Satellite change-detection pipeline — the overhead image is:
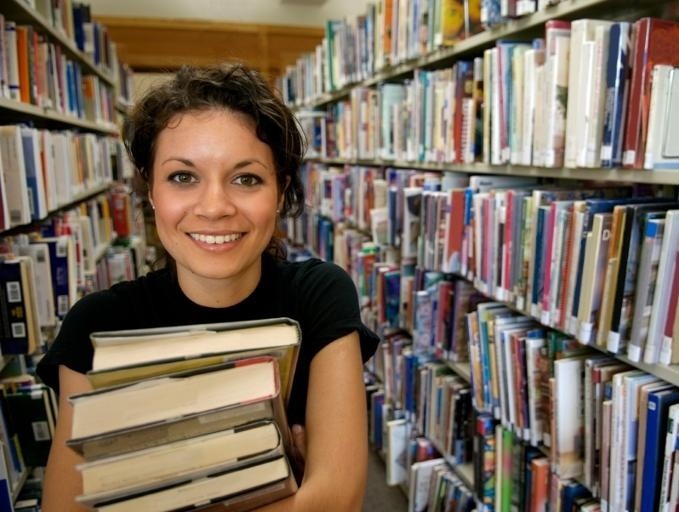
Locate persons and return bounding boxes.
[35,68,380,512]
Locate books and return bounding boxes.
[294,15,679,172]
[291,160,678,369]
[0,16,113,125]
[269,1,581,109]
[84,237,134,299]
[0,188,119,358]
[0,349,57,512]
[0,122,115,230]
[65,317,302,512]
[364,374,479,512]
[478,370,676,512]
[0,0,113,77]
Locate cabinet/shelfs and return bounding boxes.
[0,0,150,512]
[277,0,679,512]
